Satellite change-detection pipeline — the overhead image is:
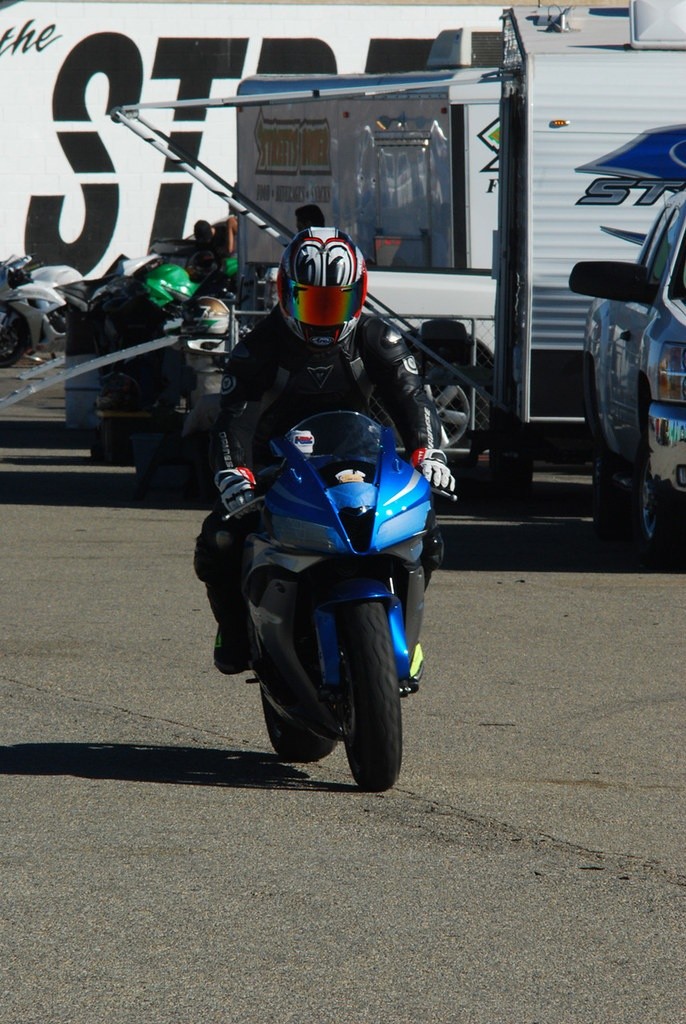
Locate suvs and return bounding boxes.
[567,189,686,557]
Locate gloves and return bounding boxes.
[215,469,261,519]
[414,448,456,494]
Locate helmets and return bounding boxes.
[277,227,368,348]
[145,263,199,306]
[191,293,231,334]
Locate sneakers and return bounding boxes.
[213,622,251,675]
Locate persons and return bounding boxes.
[195,228,456,701]
[296,203,323,233]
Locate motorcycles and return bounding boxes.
[0,254,89,369]
[230,409,434,789]
